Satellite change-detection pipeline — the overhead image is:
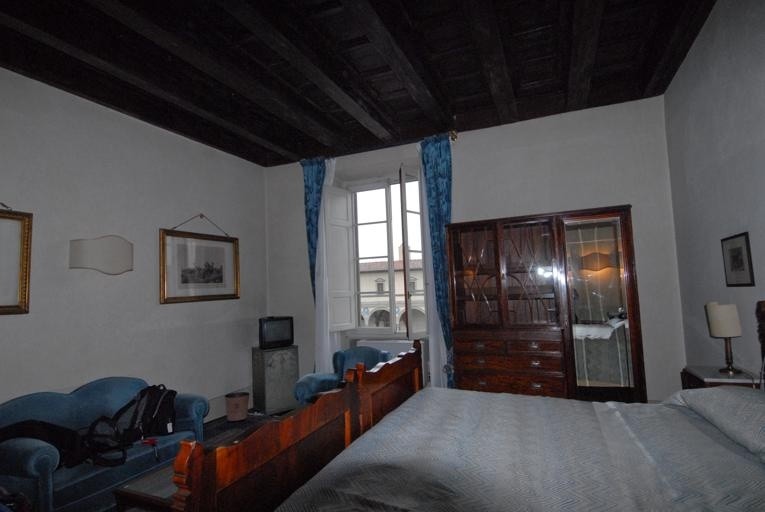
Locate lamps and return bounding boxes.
[707,302,744,374]
[68,233,135,276]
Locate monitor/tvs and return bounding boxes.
[258,315,294,351]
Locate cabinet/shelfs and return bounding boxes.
[443,201,650,404]
[252,346,300,416]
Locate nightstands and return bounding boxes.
[679,364,761,392]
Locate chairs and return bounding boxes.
[292,346,392,400]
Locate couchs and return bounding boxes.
[1,375,211,512]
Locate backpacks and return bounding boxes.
[114,384,177,436]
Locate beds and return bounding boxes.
[170,300,765,512]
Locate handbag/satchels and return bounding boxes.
[0,416,126,467]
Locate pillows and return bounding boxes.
[664,382,765,460]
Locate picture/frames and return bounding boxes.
[720,231,755,288]
[159,229,242,302]
[0,209,33,316]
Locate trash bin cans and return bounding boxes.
[225,392,249,423]
